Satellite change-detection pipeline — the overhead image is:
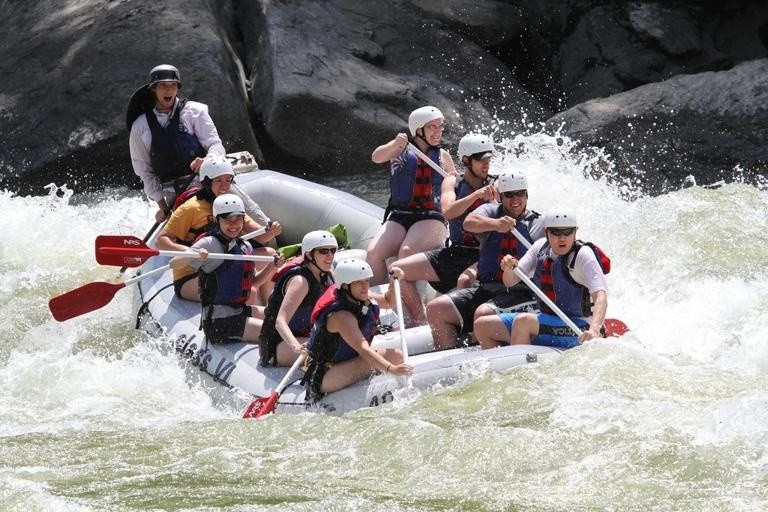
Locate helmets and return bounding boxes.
[213,194,245,220]
[498,171,528,193]
[301,230,338,261]
[334,259,374,290]
[148,64,183,90]
[543,205,577,228]
[408,106,445,138]
[459,134,494,162]
[199,156,234,182]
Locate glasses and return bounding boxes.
[425,124,444,131]
[315,248,336,255]
[503,190,526,198]
[548,228,574,236]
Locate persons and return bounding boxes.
[426,170,546,351]
[387,134,499,325]
[475,206,608,351]
[366,106,455,284]
[154,156,281,305]
[258,231,338,367]
[170,194,284,342]
[305,259,414,393]
[130,64,278,251]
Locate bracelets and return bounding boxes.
[387,363,394,373]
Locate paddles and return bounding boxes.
[243,341,305,419]
[47,228,265,322]
[95,234,277,267]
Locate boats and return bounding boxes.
[132,170,571,411]
